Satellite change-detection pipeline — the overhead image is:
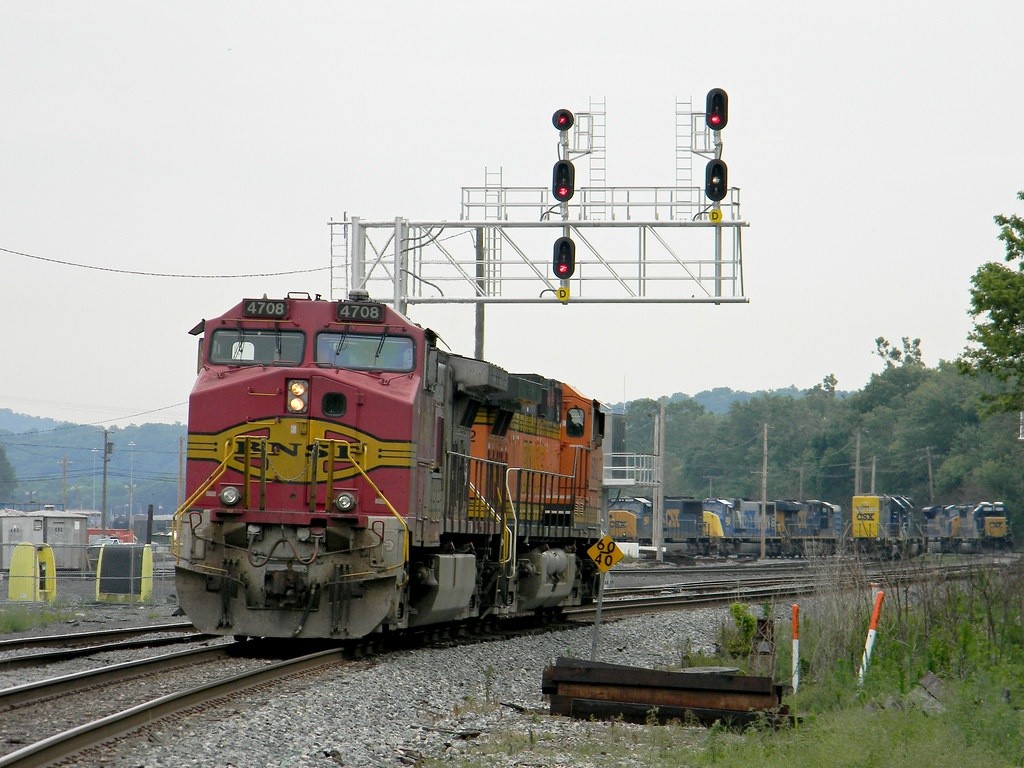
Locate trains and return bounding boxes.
[167,285,612,662]
[608,490,1011,555]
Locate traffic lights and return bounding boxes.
[551,108,574,131]
[705,87,729,132]
[552,160,575,202]
[552,237,575,279]
[706,159,728,202]
